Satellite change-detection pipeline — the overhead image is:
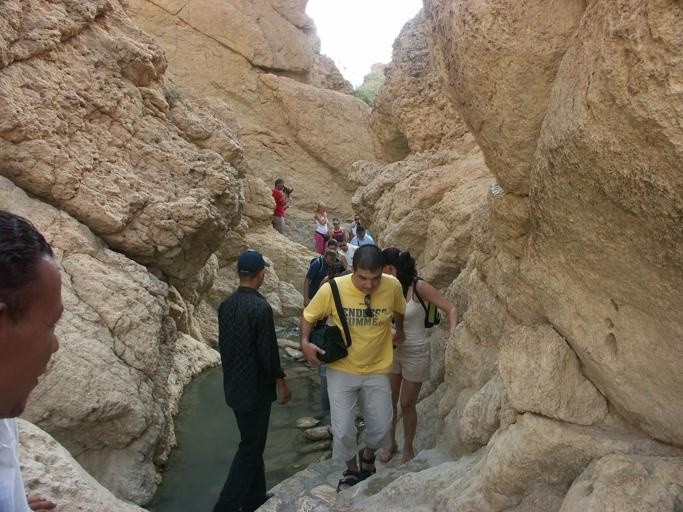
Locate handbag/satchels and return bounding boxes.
[324,235,330,241]
[424,301,441,329]
[307,324,348,365]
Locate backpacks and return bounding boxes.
[311,256,339,276]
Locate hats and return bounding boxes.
[236,250,269,276]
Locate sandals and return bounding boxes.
[401,447,413,466]
[381,440,399,463]
[358,449,378,482]
[336,466,359,497]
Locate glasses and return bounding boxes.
[364,294,373,318]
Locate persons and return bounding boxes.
[211,250,290,512]
[300,245,408,494]
[271,179,290,238]
[380,247,459,464]
[302,213,375,309]
[314,202,329,255]
[1,210,64,511]
